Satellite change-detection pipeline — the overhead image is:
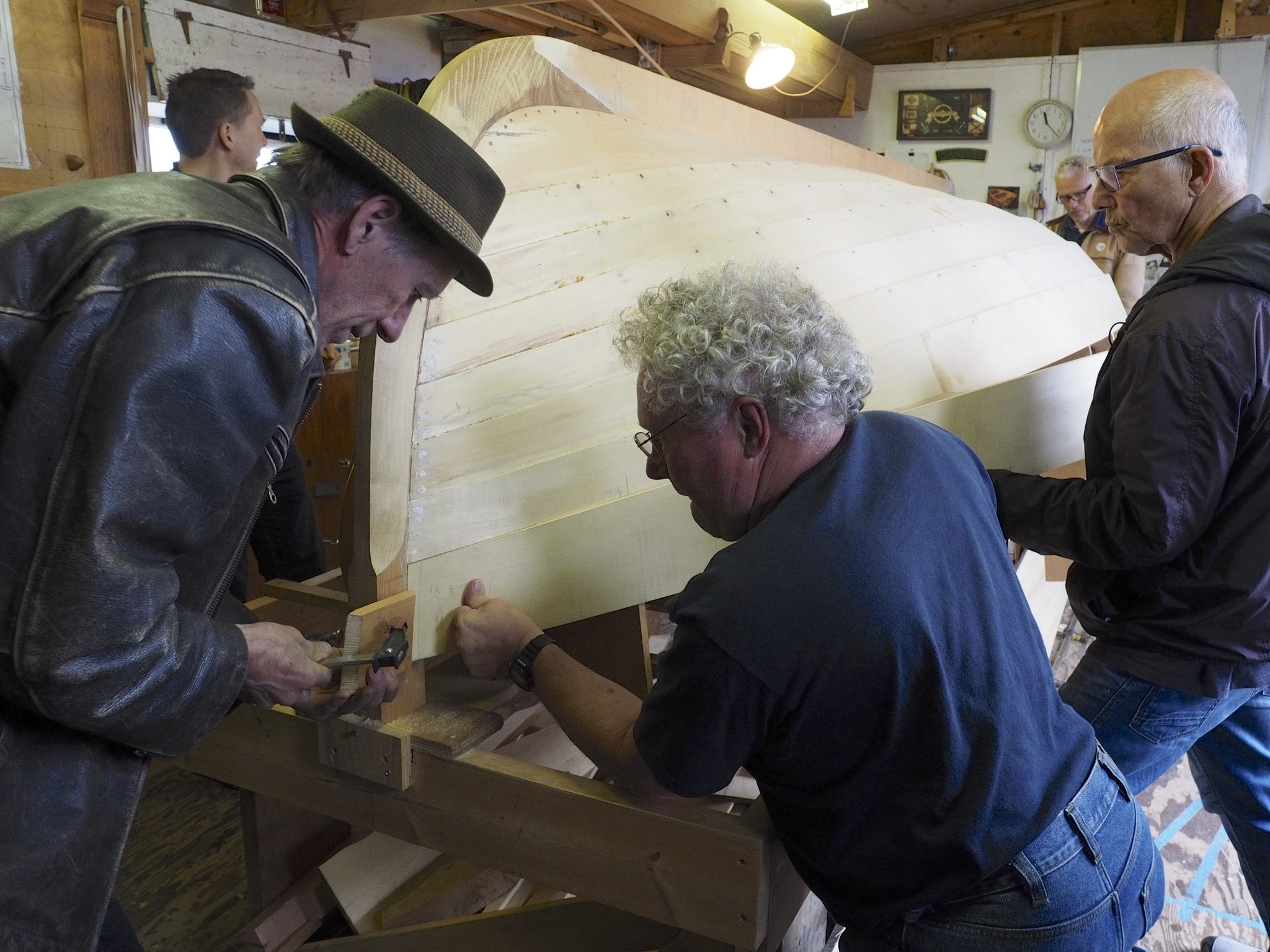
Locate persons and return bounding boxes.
[165,64,334,604]
[984,65,1270,942]
[1,91,509,951]
[1044,155,1146,317]
[447,257,1168,952]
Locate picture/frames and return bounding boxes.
[896,87,991,140]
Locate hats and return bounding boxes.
[290,86,506,298]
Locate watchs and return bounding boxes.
[508,633,561,693]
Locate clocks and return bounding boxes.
[1022,99,1072,150]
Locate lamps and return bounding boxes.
[743,32,798,90]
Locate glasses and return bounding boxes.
[1056,184,1092,204]
[1085,145,1223,193]
[634,393,759,465]
